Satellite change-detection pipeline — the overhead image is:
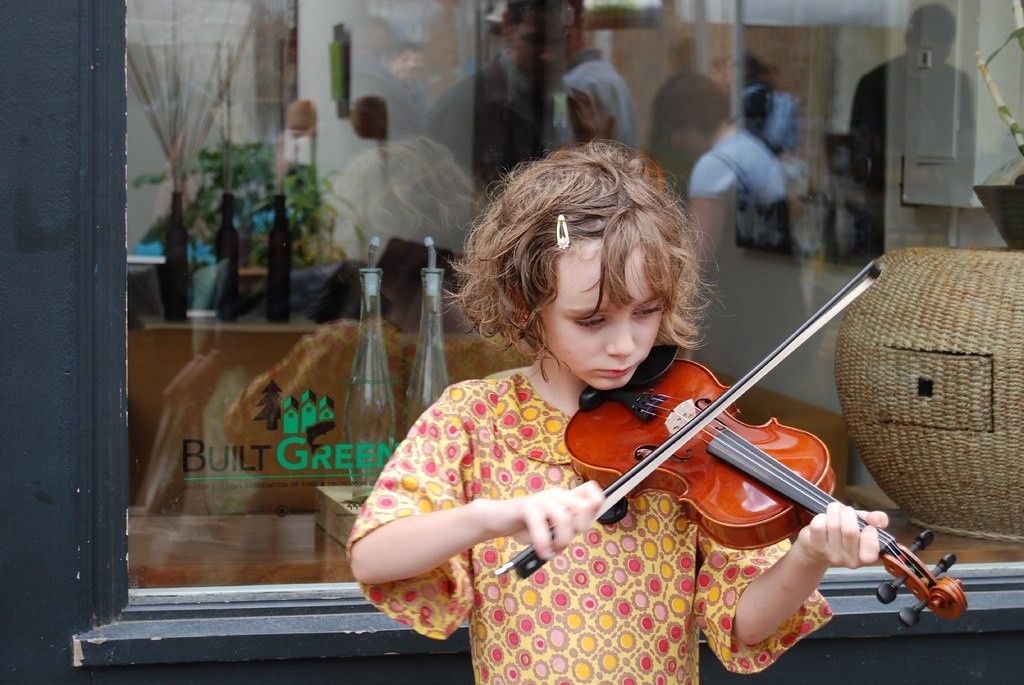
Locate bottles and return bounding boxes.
[403,237,449,438]
[342,235,396,504]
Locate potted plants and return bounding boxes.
[123,0,291,323]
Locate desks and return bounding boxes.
[129,314,351,514]
[314,486,372,560]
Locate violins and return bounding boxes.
[563,357,968,629]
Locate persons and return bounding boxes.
[844,6,975,264]
[347,139,888,685]
[346,0,805,281]
